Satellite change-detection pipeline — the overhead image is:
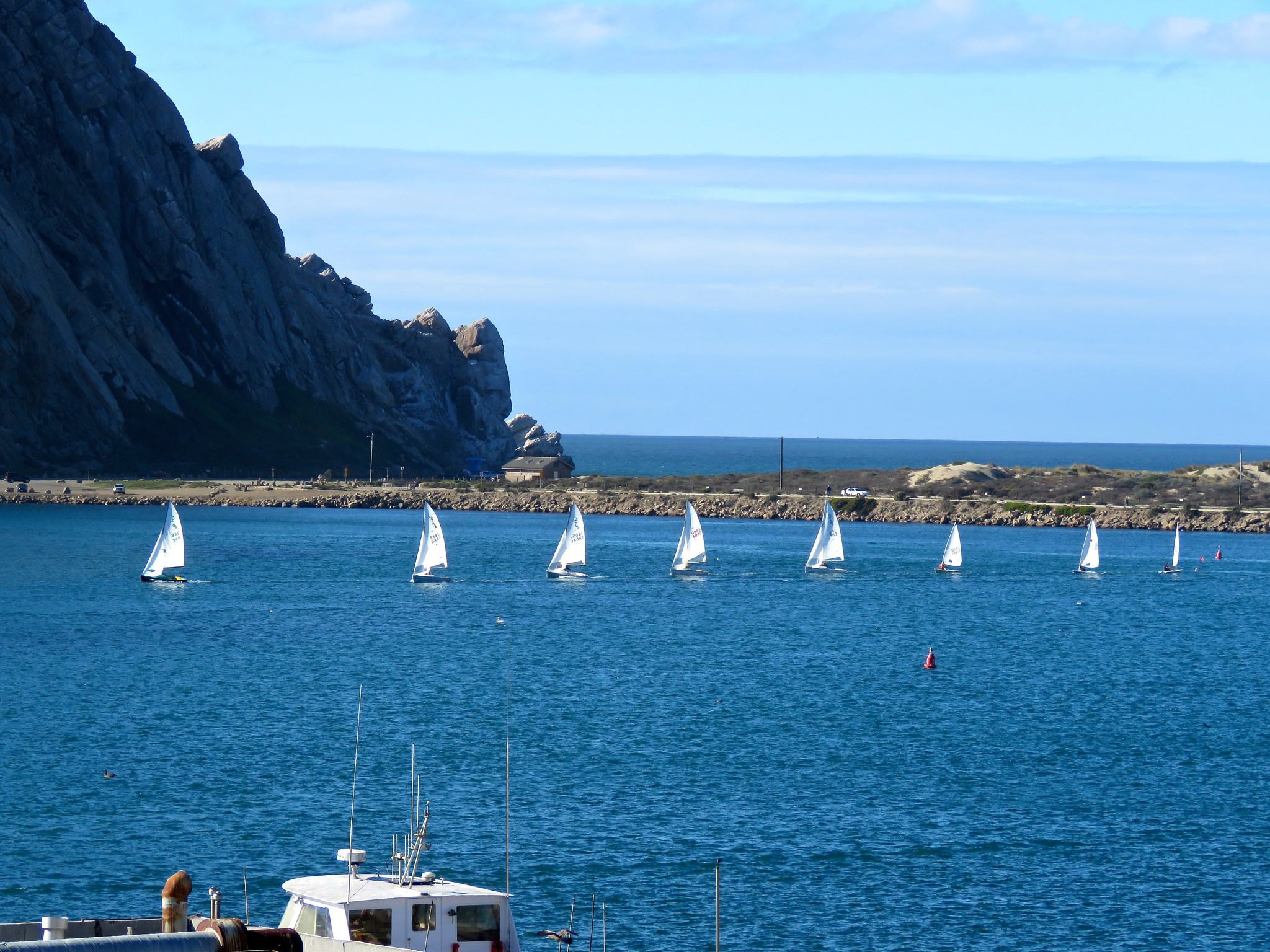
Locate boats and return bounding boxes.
[272,683,524,952]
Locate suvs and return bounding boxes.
[113,484,126,494]
[5,471,31,483]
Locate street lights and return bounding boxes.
[776,437,783,491]
[1236,448,1242,504]
[367,433,374,482]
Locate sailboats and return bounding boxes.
[934,522,962,573]
[670,499,709,576]
[805,500,847,573]
[1072,518,1106,573]
[1158,522,1183,573]
[546,502,588,578]
[412,501,452,583]
[141,501,188,582]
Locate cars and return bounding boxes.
[16,483,28,492]
[471,470,506,480]
[841,487,868,497]
